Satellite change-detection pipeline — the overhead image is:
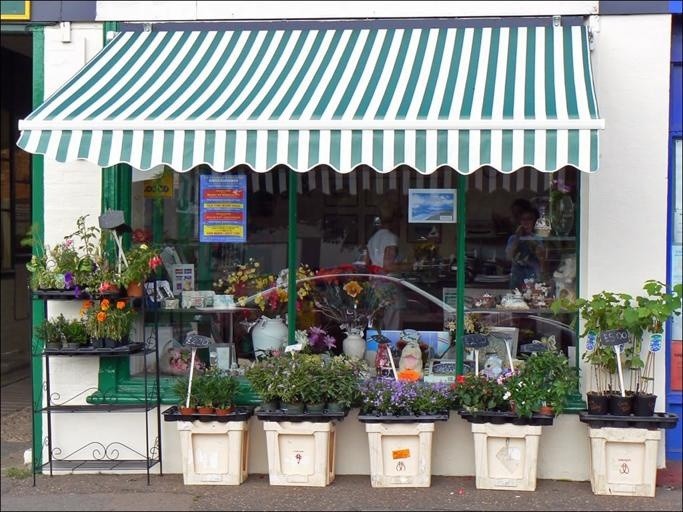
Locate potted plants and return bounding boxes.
[457,281,683,416]
[173,369,241,413]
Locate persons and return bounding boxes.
[505,198,556,291]
[365,207,419,330]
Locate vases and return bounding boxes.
[343,330,365,357]
[45,337,127,351]
[250,313,287,357]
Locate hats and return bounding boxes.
[99,211,132,233]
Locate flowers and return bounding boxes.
[211,256,395,334]
[17,215,164,343]
[246,350,452,414]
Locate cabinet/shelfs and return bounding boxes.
[32,287,162,484]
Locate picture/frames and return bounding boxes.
[318,179,444,248]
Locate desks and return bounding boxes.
[143,303,259,372]
